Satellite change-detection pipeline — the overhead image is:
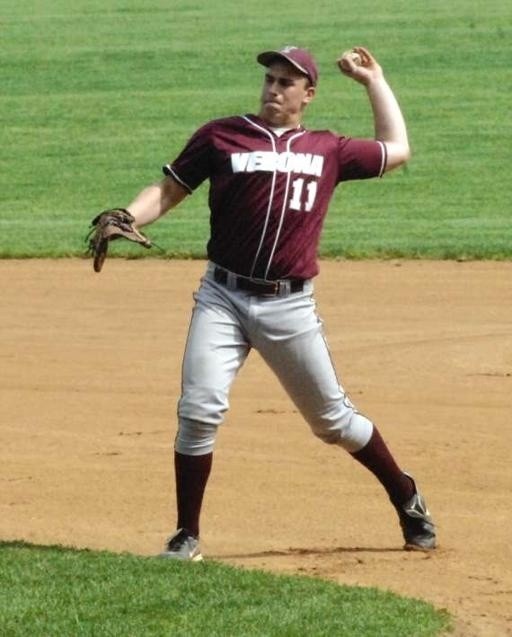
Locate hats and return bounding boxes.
[256,45,319,87]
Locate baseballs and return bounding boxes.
[338,50,365,77]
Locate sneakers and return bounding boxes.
[157,527,203,562]
[388,471,439,550]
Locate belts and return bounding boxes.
[213,266,304,297]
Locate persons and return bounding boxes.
[88,43,438,564]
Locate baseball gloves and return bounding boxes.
[86,208,152,272]
